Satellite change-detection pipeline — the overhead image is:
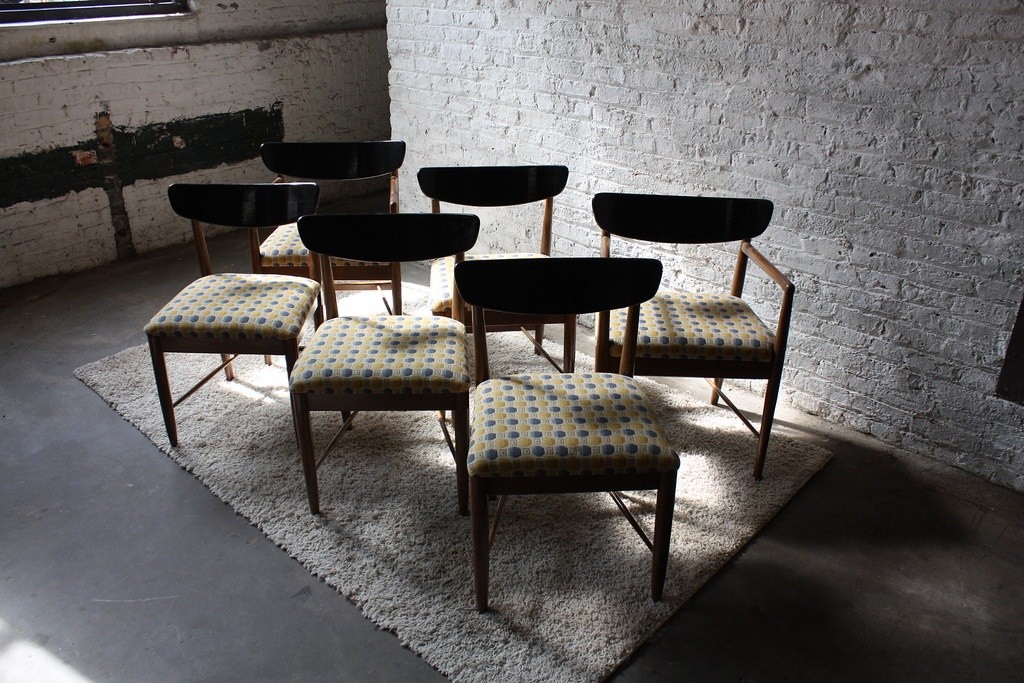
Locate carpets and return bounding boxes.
[72,273,834,683]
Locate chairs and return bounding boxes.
[590,192,795,479]
[144,182,353,453]
[289,213,480,516]
[418,164,577,376]
[249,140,406,314]
[452,257,681,613]
